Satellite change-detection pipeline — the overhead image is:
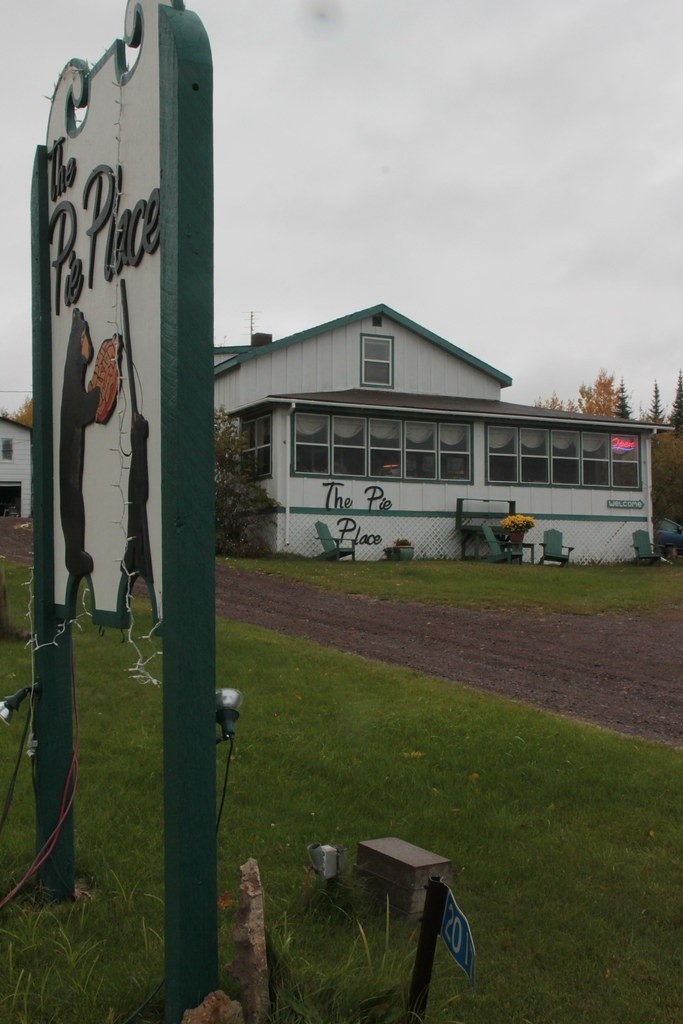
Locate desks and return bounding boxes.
[459,530,512,561]
[500,543,534,564]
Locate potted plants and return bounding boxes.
[383,538,415,560]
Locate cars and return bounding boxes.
[653,515,683,558]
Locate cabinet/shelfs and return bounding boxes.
[455,497,515,530]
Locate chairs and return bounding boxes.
[314,520,357,562]
[538,528,575,567]
[630,529,662,567]
[479,525,523,565]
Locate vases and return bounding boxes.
[508,532,524,543]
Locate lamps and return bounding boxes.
[0,681,42,726]
[382,455,400,468]
[214,687,244,740]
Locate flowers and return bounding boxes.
[500,514,536,534]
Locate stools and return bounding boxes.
[356,837,453,919]
